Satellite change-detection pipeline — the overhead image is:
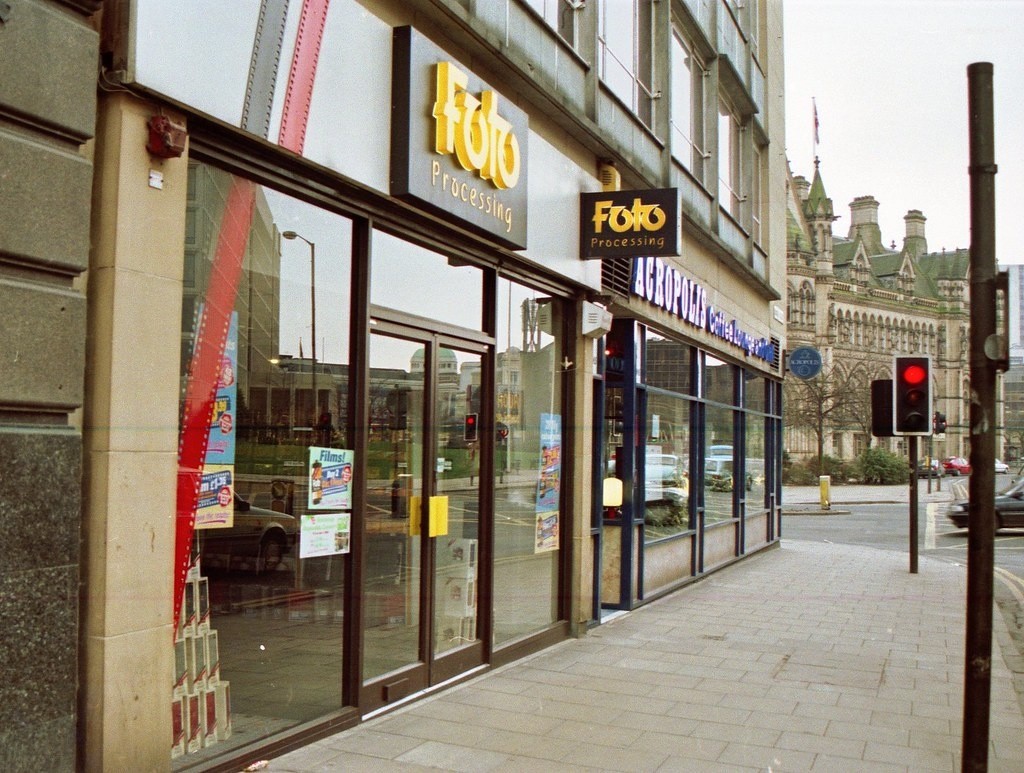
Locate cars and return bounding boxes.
[918,457,946,478]
[193,489,295,576]
[994,458,1009,473]
[941,456,971,476]
[686,445,734,483]
[607,453,678,480]
[946,465,1024,533]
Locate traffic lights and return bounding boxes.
[320,413,331,429]
[935,412,946,434]
[892,354,933,435]
[464,413,478,441]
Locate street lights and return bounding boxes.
[282,231,318,426]
